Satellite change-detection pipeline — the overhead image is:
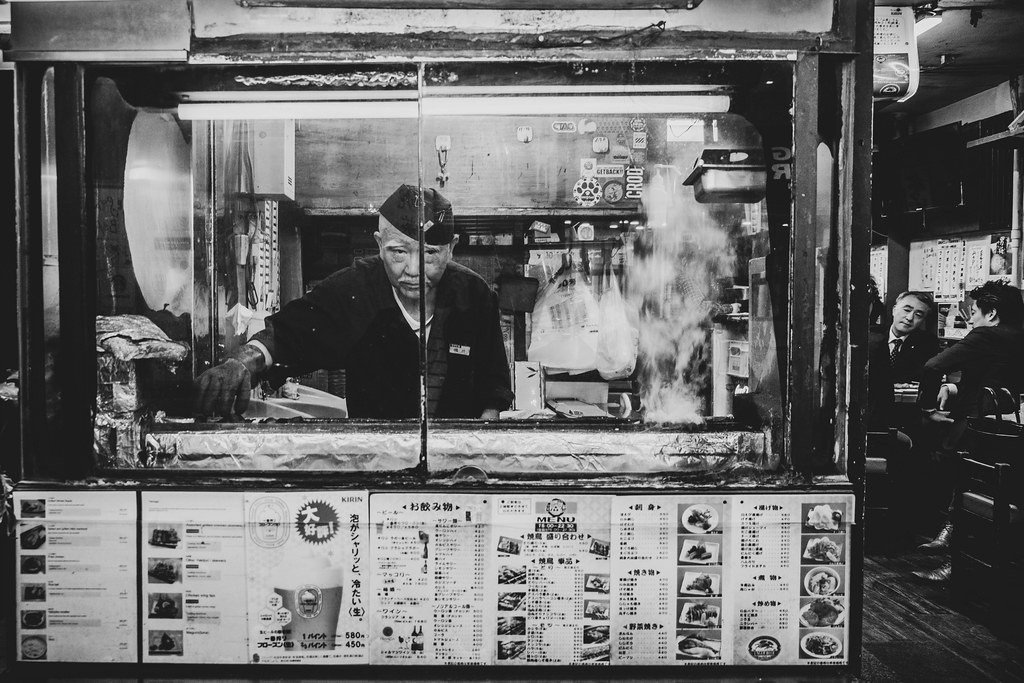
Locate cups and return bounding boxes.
[271,561,343,651]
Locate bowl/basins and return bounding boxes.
[21,636,47,659]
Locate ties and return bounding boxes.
[888,339,903,373]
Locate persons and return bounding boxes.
[190,183,516,422]
[912,284,1024,583]
[869,290,942,417]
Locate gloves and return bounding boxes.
[192,343,264,418]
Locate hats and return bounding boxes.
[379,184,454,246]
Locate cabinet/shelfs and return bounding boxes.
[521,217,642,295]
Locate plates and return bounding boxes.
[805,508,842,530]
[681,504,719,533]
[679,603,720,626]
[677,634,720,658]
[799,600,844,626]
[803,538,843,562]
[801,632,843,658]
[681,572,720,595]
[586,575,610,591]
[804,567,840,596]
[679,539,719,565]
[22,612,44,626]
[586,601,609,617]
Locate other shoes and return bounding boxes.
[917,519,953,556]
[911,560,951,587]
